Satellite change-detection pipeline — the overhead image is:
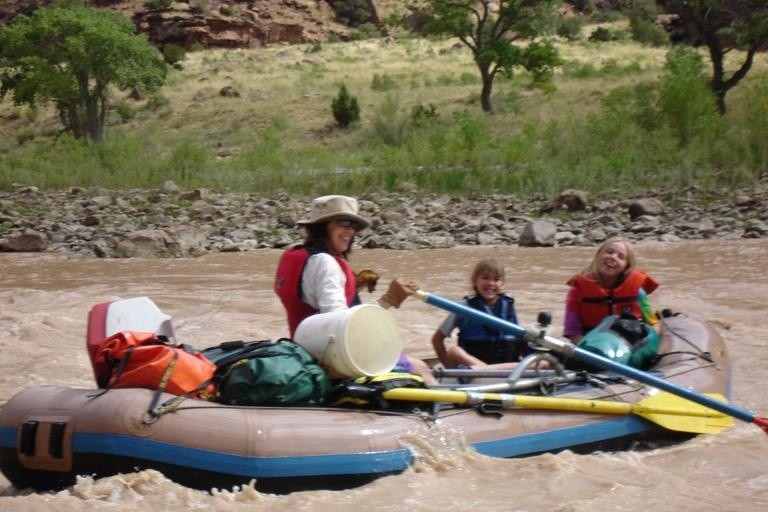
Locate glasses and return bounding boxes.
[336,219,361,230]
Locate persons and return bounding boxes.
[561,234,668,370]
[273,194,442,389]
[429,256,552,375]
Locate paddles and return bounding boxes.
[382,387,735,435]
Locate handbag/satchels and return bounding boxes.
[562,313,662,373]
[91,330,335,407]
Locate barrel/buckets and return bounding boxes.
[293,304,402,379]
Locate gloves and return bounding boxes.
[355,268,379,293]
[380,277,422,310]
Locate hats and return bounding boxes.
[293,192,370,233]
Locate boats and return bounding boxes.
[0,312,731,495]
